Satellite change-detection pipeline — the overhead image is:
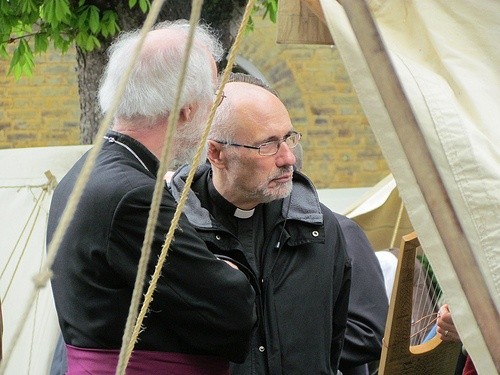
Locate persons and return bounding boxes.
[435,304,478,375]
[332,211,390,375]
[163,83,351,375]
[43,19,261,374]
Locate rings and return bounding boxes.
[444,330,449,336]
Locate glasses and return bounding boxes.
[210,89,225,107]
[208,131,303,155]
